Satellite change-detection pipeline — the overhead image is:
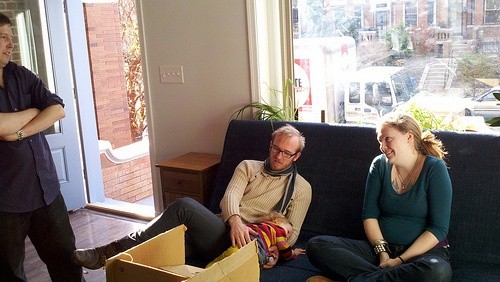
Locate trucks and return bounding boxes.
[290,35,414,125]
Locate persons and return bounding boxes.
[74,126,312,269]
[204,212,306,268]
[0,12,86,282]
[306,113,452,282]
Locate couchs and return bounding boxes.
[213,119,500,282]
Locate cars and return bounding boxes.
[462,88,500,123]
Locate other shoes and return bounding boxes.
[306,277,332,282]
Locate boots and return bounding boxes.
[75,232,142,270]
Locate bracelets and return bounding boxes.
[374,239,391,255]
[17,130,23,141]
[398,256,406,263]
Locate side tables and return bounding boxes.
[154,153,222,211]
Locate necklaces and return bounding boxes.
[396,151,419,189]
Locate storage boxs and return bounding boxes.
[106,224,260,282]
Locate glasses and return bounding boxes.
[270,144,296,158]
